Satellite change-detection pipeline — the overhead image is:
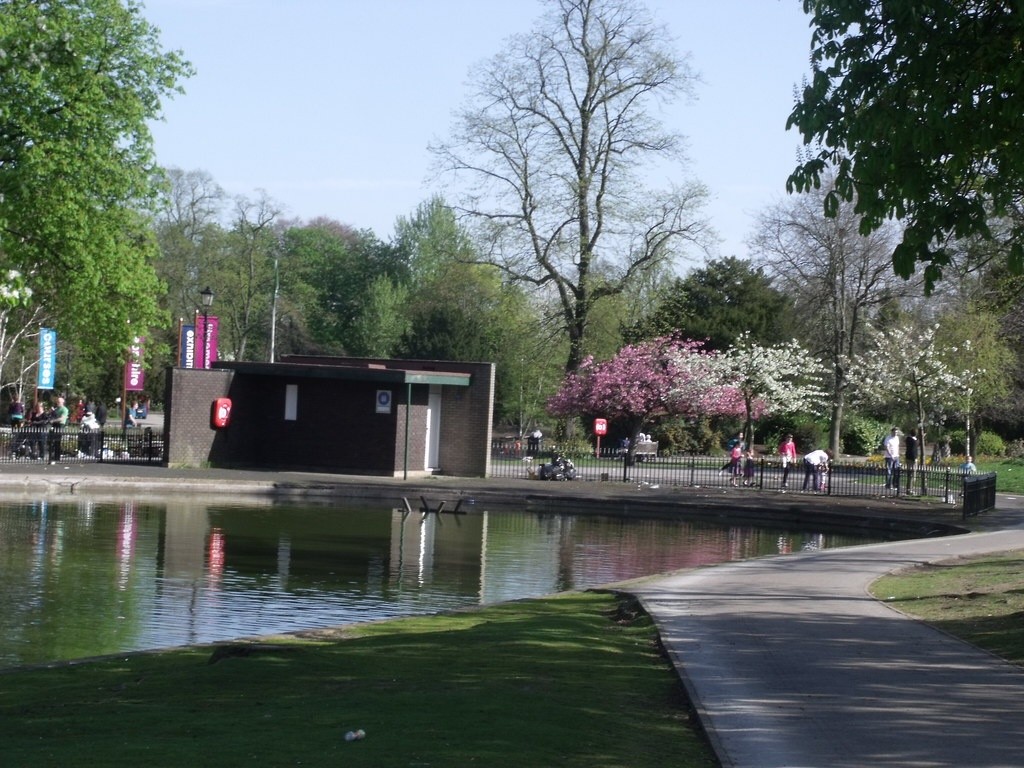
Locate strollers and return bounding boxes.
[11,424,39,461]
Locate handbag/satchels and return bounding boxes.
[781,453,788,468]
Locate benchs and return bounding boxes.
[621,440,661,462]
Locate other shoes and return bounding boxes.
[38,457,42,460]
[718,471,788,489]
[26,456,30,460]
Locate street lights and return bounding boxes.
[198,285,214,369]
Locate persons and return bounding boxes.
[9,394,107,462]
[959,456,976,496]
[802,448,832,491]
[727,440,741,486]
[719,432,745,476]
[743,451,755,487]
[883,427,901,490]
[528,429,542,451]
[818,465,829,491]
[621,433,652,462]
[777,434,796,487]
[125,400,139,428]
[905,429,919,495]
